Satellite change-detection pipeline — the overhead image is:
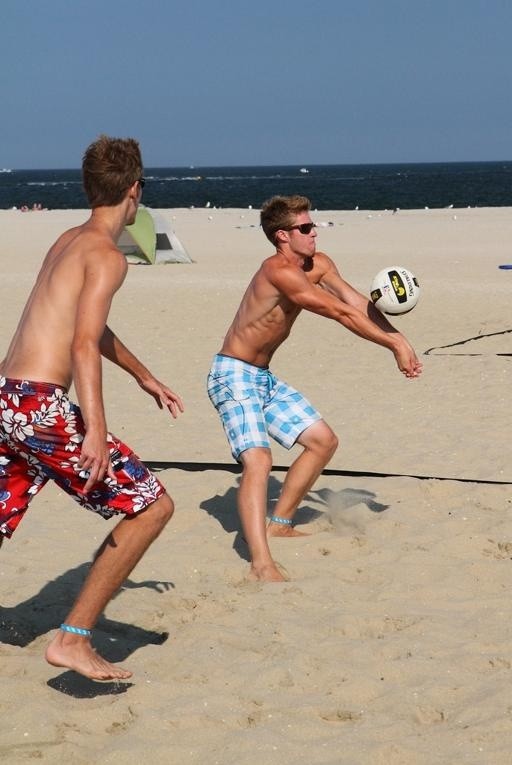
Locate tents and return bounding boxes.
[117,202,193,264]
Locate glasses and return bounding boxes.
[279,224,315,234]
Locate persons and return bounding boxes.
[208,194,425,583]
[0,134,183,679]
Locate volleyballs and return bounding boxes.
[370,266,420,315]
